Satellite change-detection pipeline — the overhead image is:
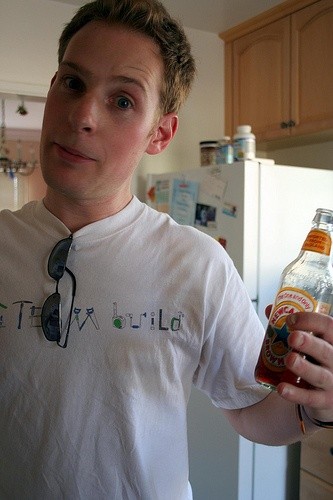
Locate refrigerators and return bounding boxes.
[146,158,333,500]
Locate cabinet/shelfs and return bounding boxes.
[299,424,333,500]
[219,0,333,151]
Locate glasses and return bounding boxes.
[40,234,76,349]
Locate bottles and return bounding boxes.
[254,208,333,395]
[199,140,217,166]
[216,136,234,164]
[233,125,257,160]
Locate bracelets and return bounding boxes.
[297,404,333,433]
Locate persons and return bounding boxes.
[0,0,333,500]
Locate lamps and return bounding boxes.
[0,97,38,178]
